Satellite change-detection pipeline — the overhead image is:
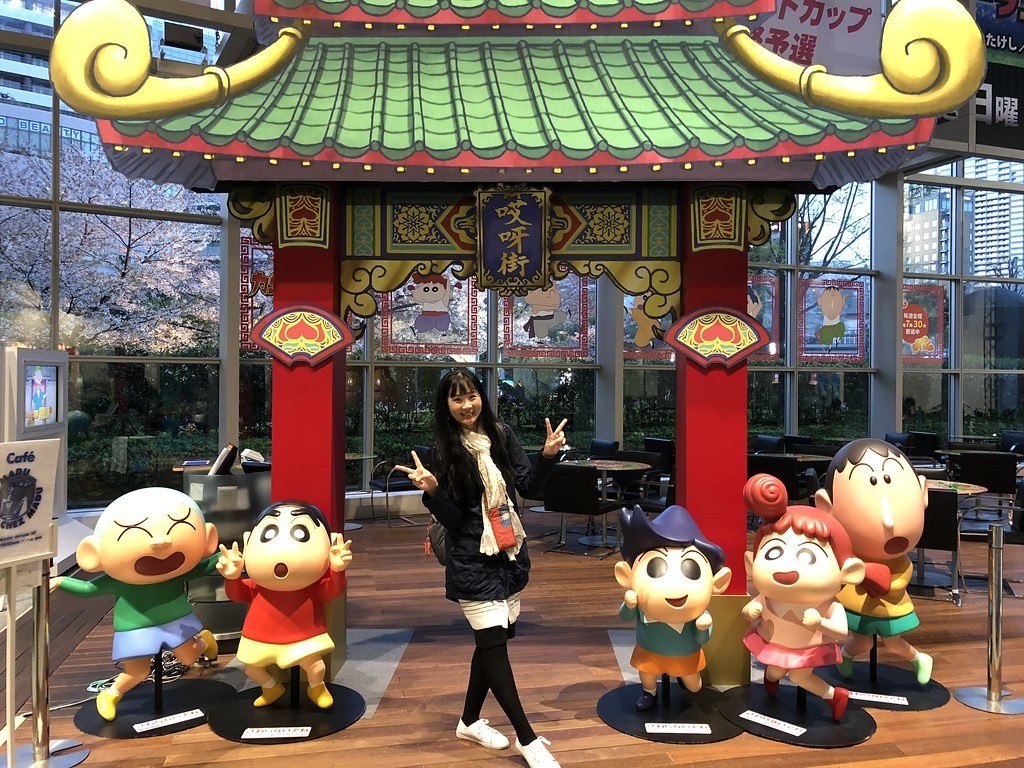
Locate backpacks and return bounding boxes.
[422,513,448,566]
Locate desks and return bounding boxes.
[758,453,833,462]
[823,438,853,445]
[344,453,378,531]
[934,450,1023,521]
[556,460,651,548]
[908,480,988,587]
[950,436,999,442]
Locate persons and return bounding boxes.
[612,503,732,715]
[394,370,572,768]
[740,437,935,725]
[49,485,245,723]
[215,498,353,708]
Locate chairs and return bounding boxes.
[526,438,676,560]
[885,431,1024,596]
[748,434,855,532]
[368,445,433,528]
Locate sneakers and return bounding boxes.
[515,736,561,768]
[456,716,509,749]
